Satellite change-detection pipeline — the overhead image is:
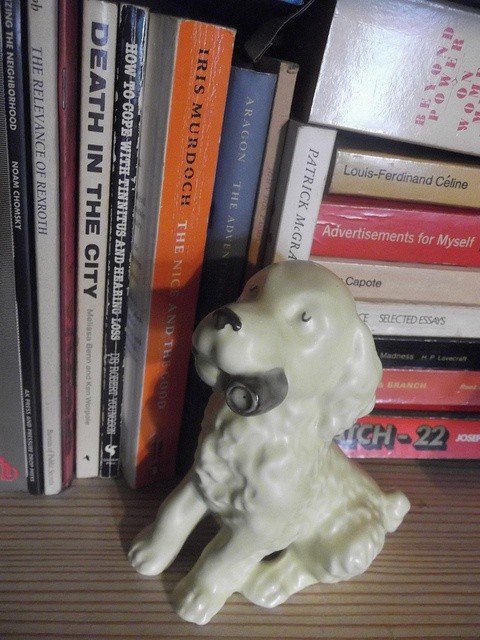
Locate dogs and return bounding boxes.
[127,260,410,624]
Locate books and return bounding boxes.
[236,52,300,301]
[176,43,278,486]
[310,257,480,304]
[371,336,480,370]
[4,0,43,494]
[354,298,480,339]
[324,131,480,209]
[310,193,480,267]
[119,12,236,490]
[308,0,479,156]
[99,3,150,479]
[58,1,83,490]
[0,0,30,492]
[258,114,337,272]
[333,409,480,460]
[28,0,62,496]
[371,368,480,412]
[75,0,118,478]
